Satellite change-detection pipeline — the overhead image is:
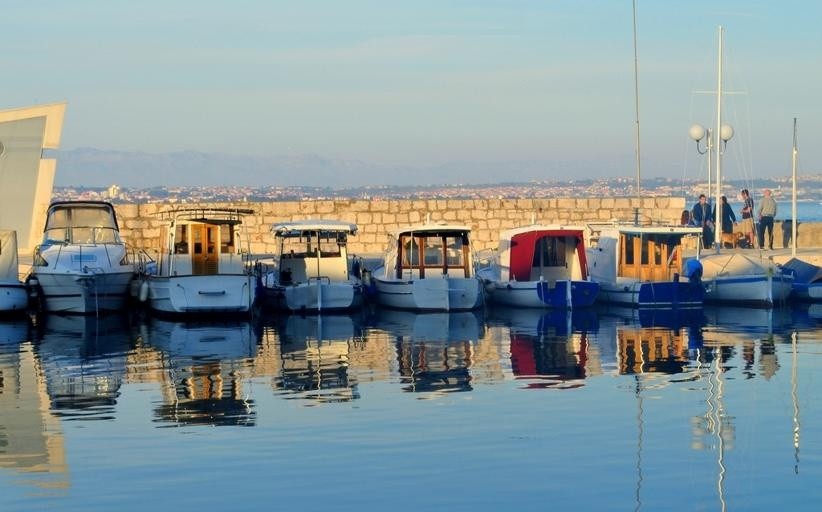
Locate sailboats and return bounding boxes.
[680,25,822,304]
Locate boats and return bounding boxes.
[260,220,384,309]
[371,225,484,310]
[32,194,136,315]
[0,230,27,311]
[476,225,600,308]
[139,203,260,312]
[586,223,708,310]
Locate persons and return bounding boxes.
[755,190,778,251]
[722,338,781,381]
[711,196,739,246]
[693,195,714,250]
[681,209,696,235]
[739,189,754,250]
